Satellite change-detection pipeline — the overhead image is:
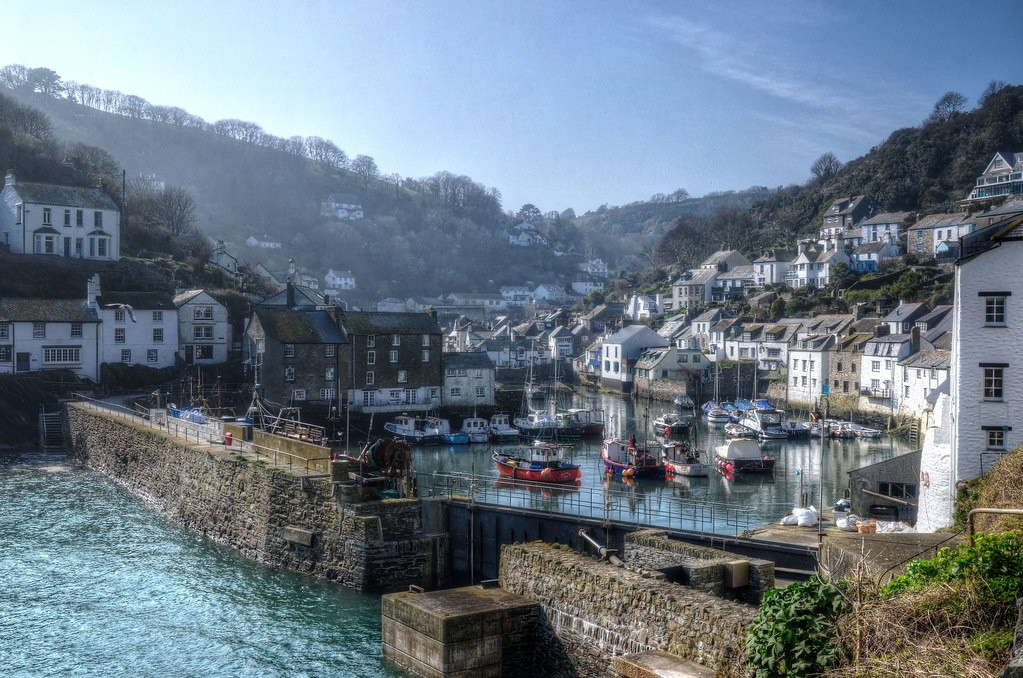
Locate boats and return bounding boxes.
[599,407,664,481]
[603,467,667,516]
[491,441,582,485]
[652,407,699,439]
[700,345,884,441]
[714,437,778,475]
[673,394,695,411]
[664,470,710,494]
[659,439,712,478]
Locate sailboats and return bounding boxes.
[168,332,608,448]
